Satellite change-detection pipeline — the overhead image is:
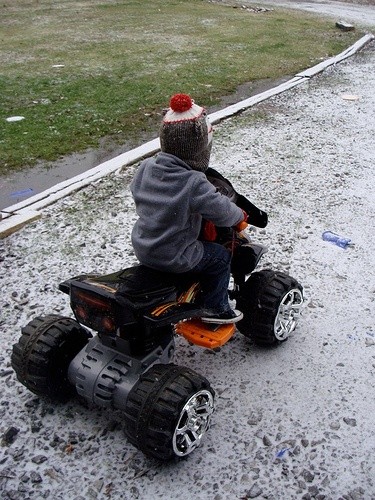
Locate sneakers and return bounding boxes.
[199,306,244,325]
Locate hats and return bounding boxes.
[159,93,214,172]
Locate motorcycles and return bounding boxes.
[9,167,305,468]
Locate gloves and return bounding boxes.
[231,206,248,233]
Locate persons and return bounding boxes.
[129,93,249,324]
